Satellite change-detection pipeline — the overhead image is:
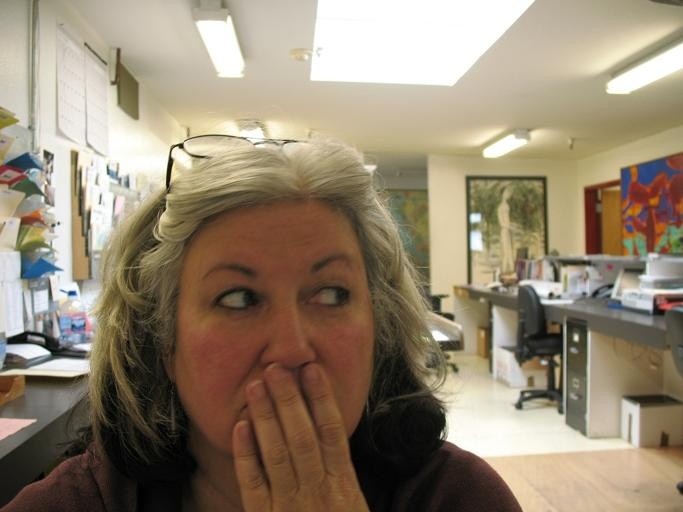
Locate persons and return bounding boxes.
[1,140,523,512]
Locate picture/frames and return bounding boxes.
[465,176,550,283]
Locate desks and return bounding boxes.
[453,272,672,439]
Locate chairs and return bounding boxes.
[505,282,568,416]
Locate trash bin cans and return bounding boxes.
[623,396,683,447]
[497,346,548,384]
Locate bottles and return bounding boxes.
[59,290,87,344]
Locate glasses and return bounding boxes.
[166,134,312,193]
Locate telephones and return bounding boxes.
[7,331,60,352]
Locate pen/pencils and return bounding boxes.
[607,302,624,309]
